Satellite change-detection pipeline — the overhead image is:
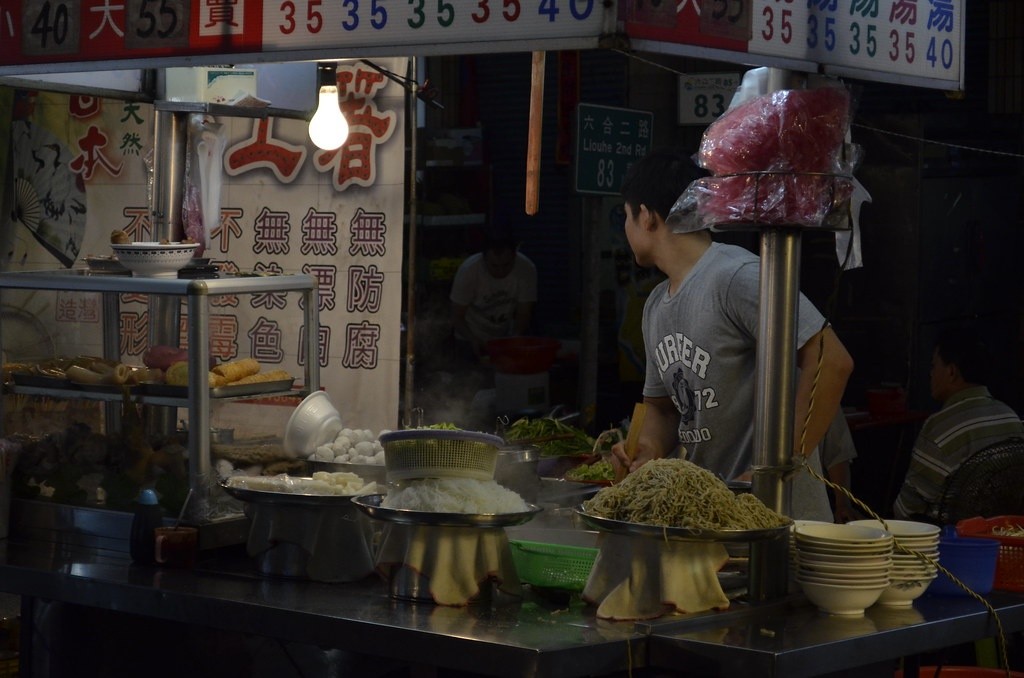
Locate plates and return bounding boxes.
[180,267,219,273]
[217,482,389,506]
[351,492,546,527]
[572,504,794,542]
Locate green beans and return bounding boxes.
[505,415,614,458]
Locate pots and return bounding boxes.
[176,427,235,445]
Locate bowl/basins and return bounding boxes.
[542,452,600,476]
[80,255,128,270]
[110,241,199,276]
[789,521,940,614]
[283,389,343,454]
[496,450,559,481]
[188,257,216,265]
[303,458,387,479]
[716,478,754,598]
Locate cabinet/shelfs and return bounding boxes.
[0,264,321,550]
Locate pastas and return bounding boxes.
[583,456,794,552]
[987,523,1024,564]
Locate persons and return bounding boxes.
[622,149,852,524]
[449,233,537,362]
[893,327,1024,522]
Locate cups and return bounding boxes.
[155,527,197,563]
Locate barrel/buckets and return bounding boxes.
[893,666,1024,678]
[379,564,486,606]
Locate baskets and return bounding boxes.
[509,536,599,587]
[379,428,503,482]
[954,511,1024,590]
[487,336,563,375]
